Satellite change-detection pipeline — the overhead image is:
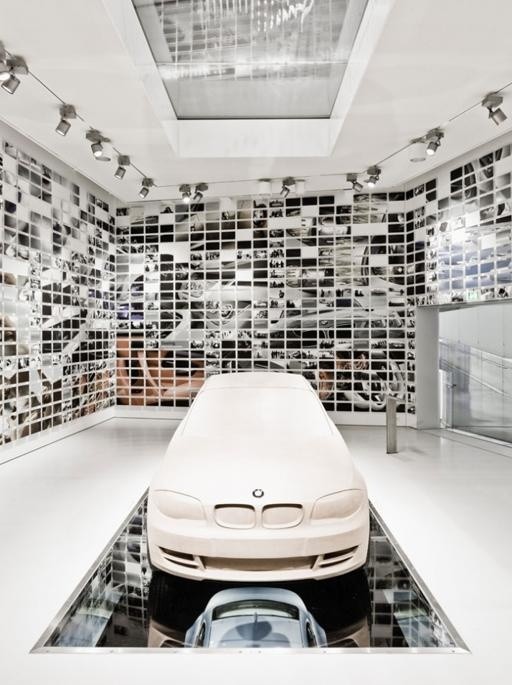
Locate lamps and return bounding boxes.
[174,173,364,203]
[0,40,157,200]
[364,92,508,190]
